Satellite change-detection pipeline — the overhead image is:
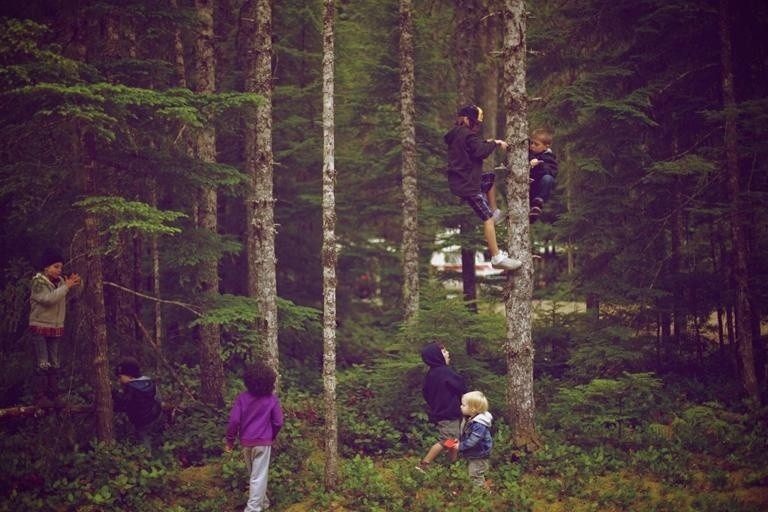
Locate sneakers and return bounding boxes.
[530,200,542,216]
[491,255,524,272]
[414,459,428,474]
[492,209,508,226]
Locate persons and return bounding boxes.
[447,104,522,272]
[108,359,167,459]
[27,246,83,409]
[222,368,284,512]
[454,386,493,487]
[416,339,464,469]
[529,128,557,221]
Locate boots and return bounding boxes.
[47,375,68,408]
[31,375,54,408]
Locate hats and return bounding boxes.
[455,104,485,123]
[40,246,64,270]
